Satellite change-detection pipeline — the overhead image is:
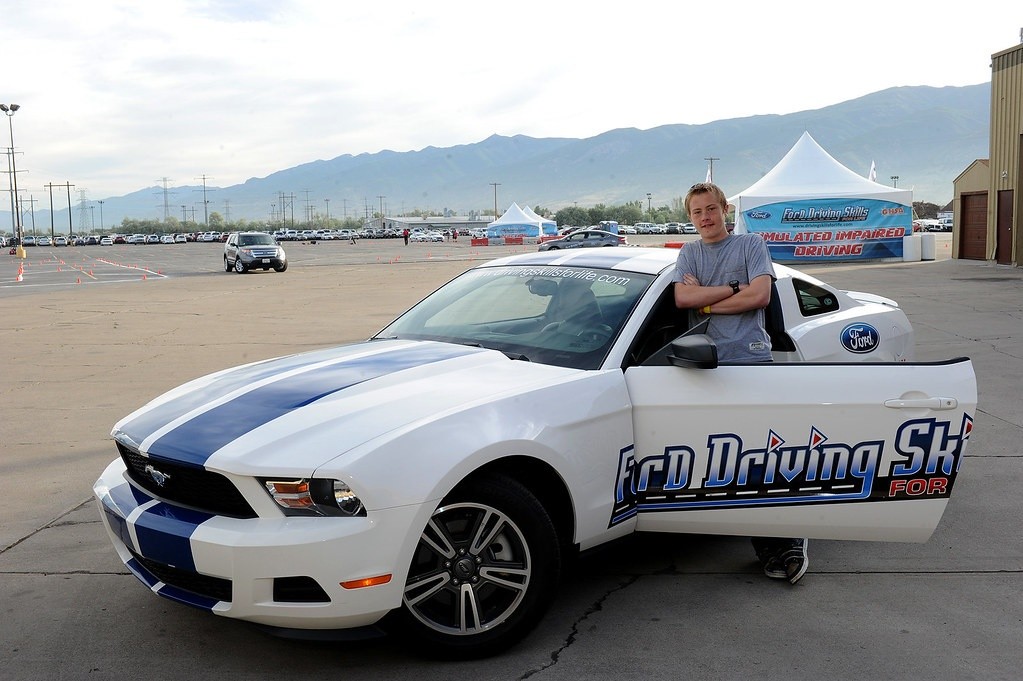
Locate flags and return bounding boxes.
[705,168,711,183]
[868,161,876,181]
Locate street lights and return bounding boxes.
[646,192,654,215]
[890,175,899,189]
[488,182,503,220]
[376,195,389,229]
[323,199,332,220]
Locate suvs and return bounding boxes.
[222,230,288,273]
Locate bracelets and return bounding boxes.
[704,305,710,314]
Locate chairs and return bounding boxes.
[651,280,690,347]
[546,277,602,326]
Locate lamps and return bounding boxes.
[1002,170,1008,177]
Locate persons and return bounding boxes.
[453,230,458,242]
[672,182,809,584]
[403,228,408,245]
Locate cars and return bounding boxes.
[147,235,159,244]
[89,241,979,658]
[559,221,738,236]
[0,234,148,249]
[472,228,488,238]
[409,233,429,244]
[221,232,230,242]
[425,232,445,243]
[160,231,214,244]
[537,229,628,254]
[914,212,952,234]
[233,228,472,241]
[212,232,221,241]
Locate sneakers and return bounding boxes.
[756,545,788,578]
[780,537,809,584]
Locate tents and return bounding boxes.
[722,131,914,263]
[488,202,557,237]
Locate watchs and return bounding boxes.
[729,280,740,293]
